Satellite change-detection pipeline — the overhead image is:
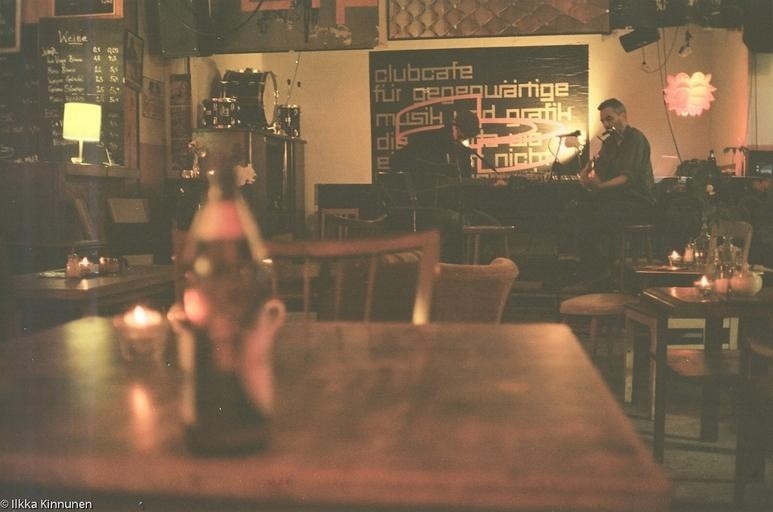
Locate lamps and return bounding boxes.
[62,102,102,164]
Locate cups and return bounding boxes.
[99,256,119,273]
[668,255,681,268]
[694,281,713,303]
[112,314,165,366]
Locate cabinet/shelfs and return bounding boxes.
[192,127,307,240]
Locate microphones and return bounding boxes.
[559,130,581,137]
[679,32,692,57]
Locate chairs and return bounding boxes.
[0,206,771,511]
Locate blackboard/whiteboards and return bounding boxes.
[0,23,38,163]
[37,15,127,167]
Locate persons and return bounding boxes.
[559,97,656,267]
[392,109,515,266]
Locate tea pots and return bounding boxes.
[66,247,82,279]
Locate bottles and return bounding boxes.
[728,149,742,177]
[708,150,716,174]
[713,264,730,294]
[180,158,275,458]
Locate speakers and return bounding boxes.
[619,27,661,53]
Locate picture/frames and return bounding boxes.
[48,0,124,19]
[125,28,145,92]
[0,0,22,52]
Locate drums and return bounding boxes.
[278,104,300,137]
[202,98,241,129]
[220,72,277,127]
